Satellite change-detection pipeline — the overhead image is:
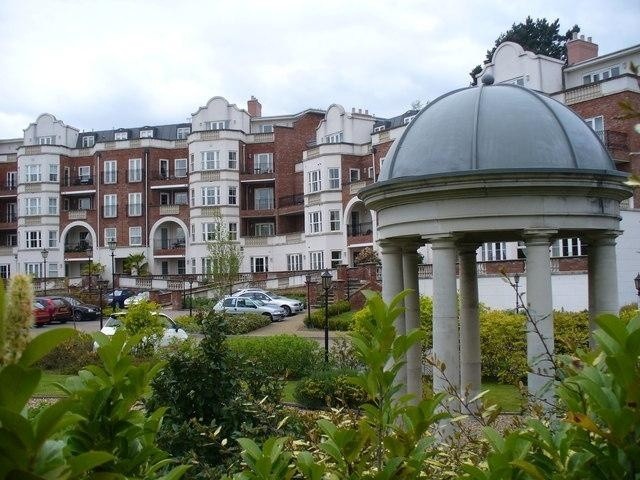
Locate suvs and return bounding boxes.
[91,310,188,357]
[37,297,73,324]
[103,287,134,309]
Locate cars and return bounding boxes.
[61,296,99,323]
[241,290,305,318]
[231,287,266,299]
[33,302,50,328]
[210,295,285,323]
[123,291,150,310]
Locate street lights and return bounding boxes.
[187,273,197,317]
[96,274,105,330]
[304,271,312,321]
[40,247,50,295]
[346,265,351,300]
[320,267,334,363]
[86,245,93,298]
[107,236,118,313]
[633,271,640,297]
[513,271,521,313]
